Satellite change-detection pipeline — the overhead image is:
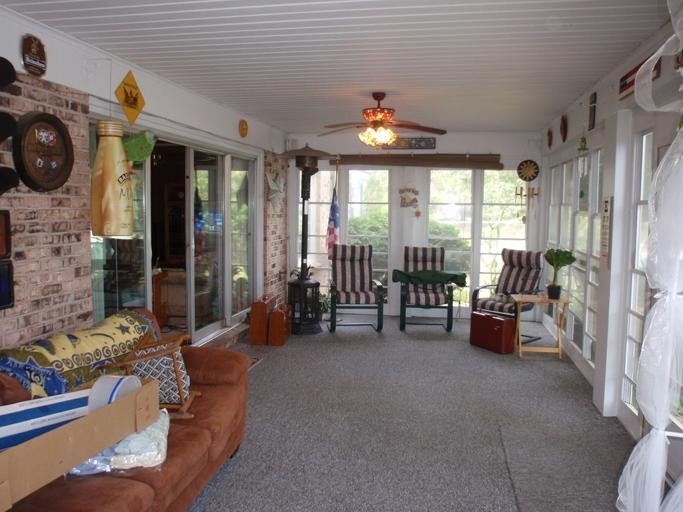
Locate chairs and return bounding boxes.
[327,244,543,348]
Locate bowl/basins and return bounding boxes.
[87,373,141,410]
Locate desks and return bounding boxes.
[510,293,574,360]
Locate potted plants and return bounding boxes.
[543,247,576,299]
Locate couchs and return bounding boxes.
[2,309,254,511]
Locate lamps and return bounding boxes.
[357,123,399,148]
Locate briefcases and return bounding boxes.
[251,294,291,346]
[470,311,516,354]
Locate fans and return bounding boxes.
[315,91,448,139]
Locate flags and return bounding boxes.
[326,185,339,260]
